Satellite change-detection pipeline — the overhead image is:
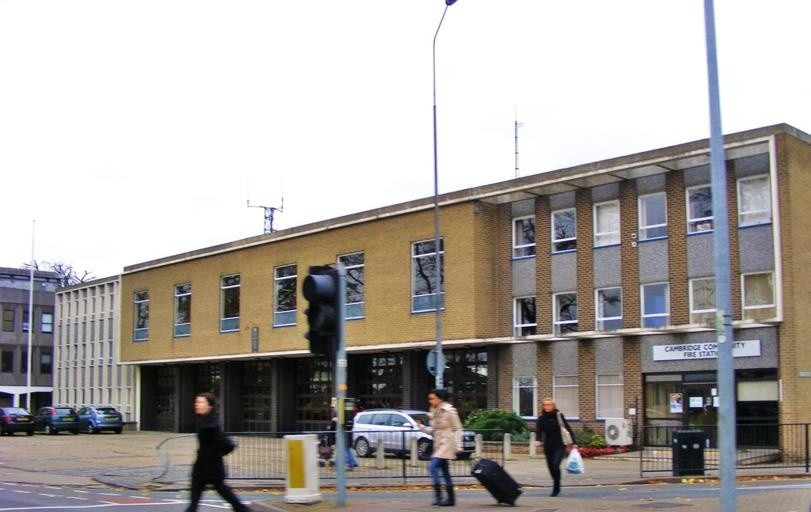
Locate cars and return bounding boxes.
[351,408,477,460]
[33,406,78,434]
[78,404,123,433]
[0,406,33,436]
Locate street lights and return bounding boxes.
[435,1,457,390]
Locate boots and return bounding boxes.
[432,484,455,505]
[550,469,560,496]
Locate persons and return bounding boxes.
[414,390,466,508]
[534,396,579,499]
[330,416,360,468]
[183,390,253,512]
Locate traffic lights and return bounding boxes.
[303,264,337,357]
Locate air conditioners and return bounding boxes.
[605,419,634,447]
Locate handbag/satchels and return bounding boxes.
[560,426,574,446]
[218,430,234,456]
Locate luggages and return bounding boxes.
[456,452,521,506]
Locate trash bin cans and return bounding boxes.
[672,431,704,477]
[284,434,323,504]
